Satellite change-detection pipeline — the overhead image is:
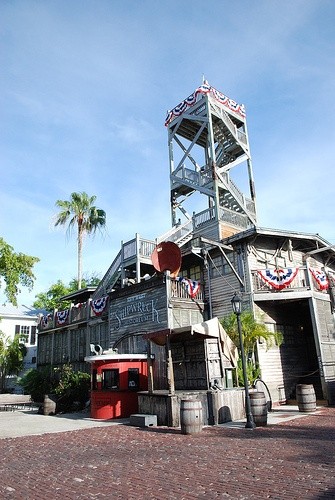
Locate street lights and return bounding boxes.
[231,291,256,428]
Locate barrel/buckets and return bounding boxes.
[296,384,316,411]
[181,400,202,433]
[245,392,267,426]
[44,394,56,416]
